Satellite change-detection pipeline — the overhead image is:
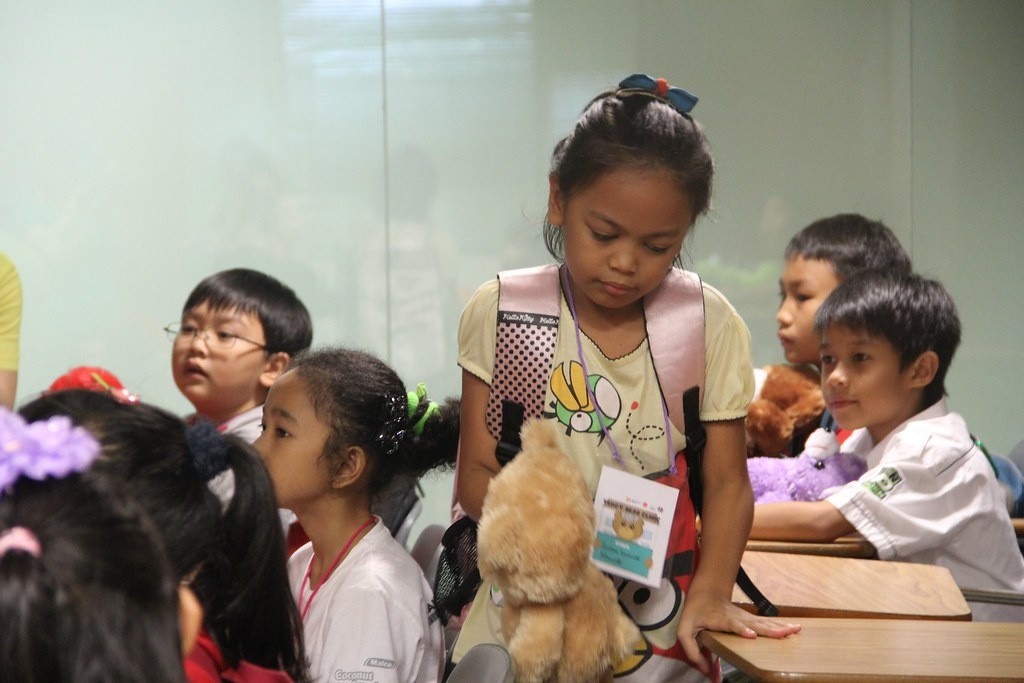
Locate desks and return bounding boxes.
[692,614,1024,683]
[734,549,972,624]
[744,526,874,558]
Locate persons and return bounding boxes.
[776,212,912,448]
[251,348,462,683]
[0,390,307,683]
[163,268,313,546]
[0,252,23,413]
[456,75,801,683]
[694,269,1023,622]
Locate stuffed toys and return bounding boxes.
[742,362,826,458]
[478,416,641,683]
[744,428,866,505]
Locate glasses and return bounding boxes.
[164,319,270,349]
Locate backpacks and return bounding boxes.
[433,264,778,637]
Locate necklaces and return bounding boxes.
[297,516,376,625]
